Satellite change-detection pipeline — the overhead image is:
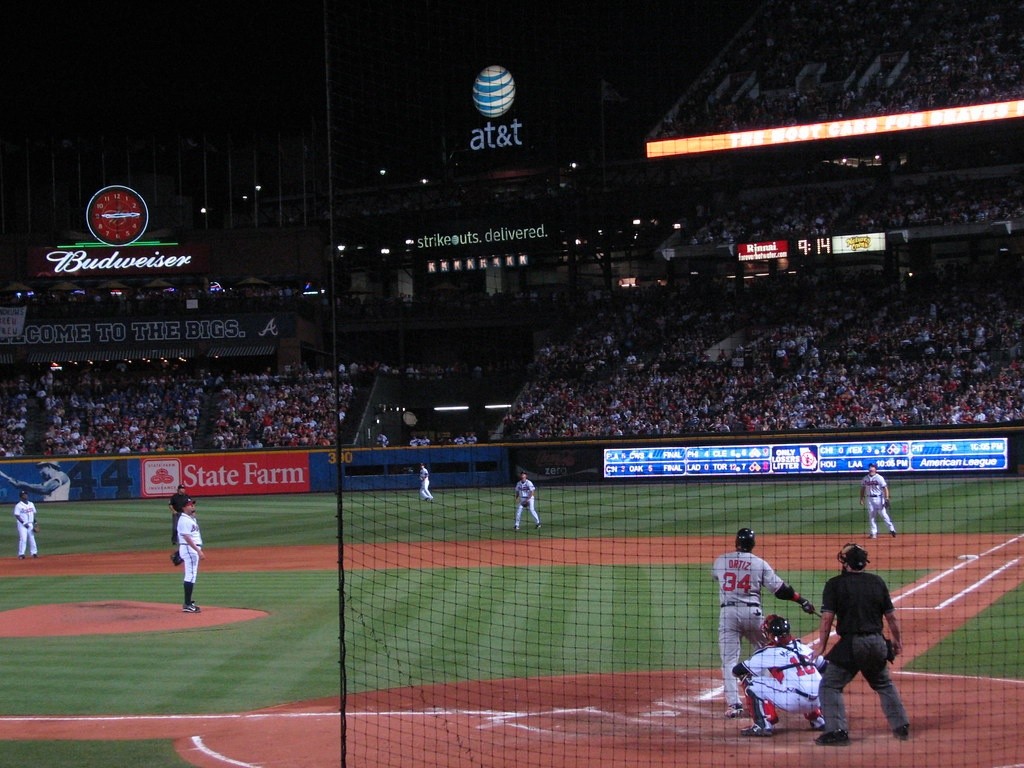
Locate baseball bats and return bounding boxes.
[798,604,839,628]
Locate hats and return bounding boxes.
[179,498,196,509]
[845,548,868,566]
[19,490,28,496]
[177,484,186,488]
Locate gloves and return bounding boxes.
[801,601,816,614]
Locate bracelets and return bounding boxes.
[34,522,38,524]
[23,522,26,525]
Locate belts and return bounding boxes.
[795,689,810,698]
[720,601,759,607]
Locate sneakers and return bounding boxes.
[183,601,200,612]
[893,725,909,740]
[741,723,773,736]
[724,703,744,716]
[814,729,852,746]
[810,717,825,730]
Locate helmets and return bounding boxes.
[769,618,791,637]
[735,528,756,551]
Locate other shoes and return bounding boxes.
[891,530,897,538]
[512,526,519,530]
[868,535,876,539]
[19,555,25,560]
[32,554,40,559]
[535,524,541,529]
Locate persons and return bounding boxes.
[421,466,433,503]
[8,459,70,502]
[676,171,1024,247]
[807,543,910,746]
[512,470,542,530]
[495,251,1024,438]
[860,462,898,539]
[0,278,483,450]
[711,529,816,718]
[654,0,1024,153]
[168,483,192,545]
[177,497,206,614]
[13,491,41,559]
[730,613,829,736]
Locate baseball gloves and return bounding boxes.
[882,498,890,508]
[170,550,184,566]
[522,500,530,507]
